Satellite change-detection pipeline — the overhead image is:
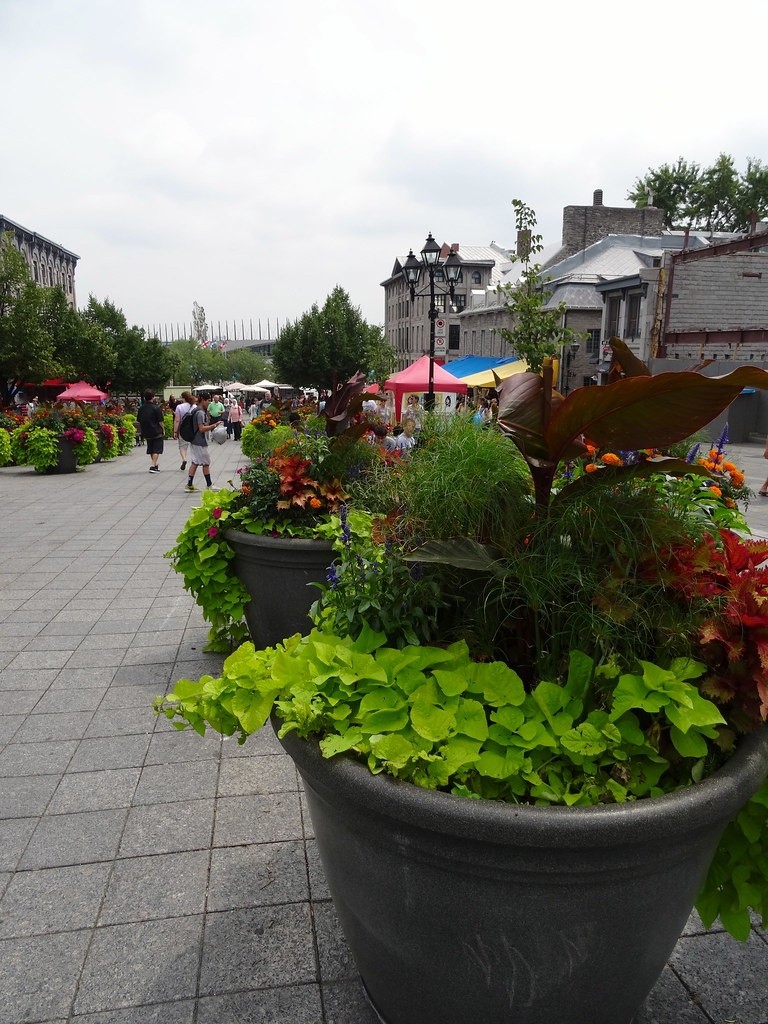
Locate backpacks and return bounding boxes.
[180,409,206,442]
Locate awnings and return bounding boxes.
[441,354,558,389]
[192,379,295,402]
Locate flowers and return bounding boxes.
[551,339,756,537]
[99,406,137,457]
[11,408,100,475]
[163,369,387,655]
[82,405,124,460]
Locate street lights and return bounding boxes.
[401,231,462,414]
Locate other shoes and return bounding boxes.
[149,464,161,473]
[180,459,187,470]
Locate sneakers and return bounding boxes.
[206,483,222,492]
[184,484,201,492]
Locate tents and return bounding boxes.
[56,381,108,403]
[15,377,96,401]
[384,355,467,426]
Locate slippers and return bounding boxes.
[759,491,768,497]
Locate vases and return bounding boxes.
[94,431,103,463]
[44,437,78,474]
[225,527,341,651]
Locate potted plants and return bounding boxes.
[150,200,768,1024]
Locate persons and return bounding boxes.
[444,396,451,407]
[109,396,168,415]
[407,396,412,404]
[26,396,39,419]
[758,433,768,497]
[455,387,499,430]
[137,389,166,474]
[167,389,420,493]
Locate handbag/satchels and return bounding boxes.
[240,421,247,428]
[211,422,228,445]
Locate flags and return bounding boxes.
[193,339,227,351]
